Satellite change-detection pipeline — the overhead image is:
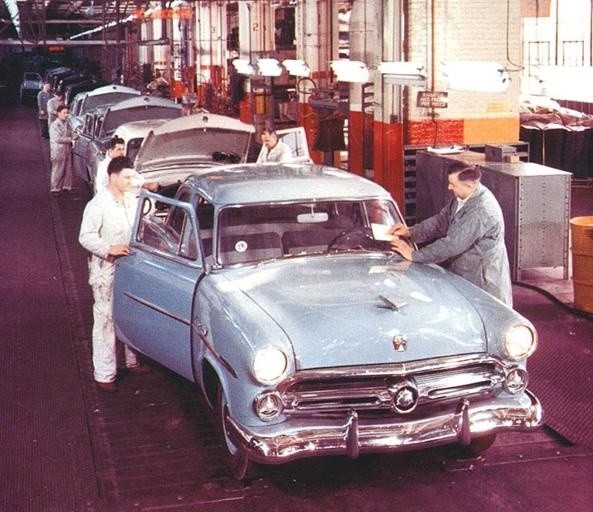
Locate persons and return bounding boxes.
[255,127,294,164]
[389,160,515,310]
[38,82,72,196]
[146,77,170,98]
[76,155,159,394]
[94,137,125,193]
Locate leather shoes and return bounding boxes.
[128,364,150,374]
[51,190,73,196]
[96,381,119,393]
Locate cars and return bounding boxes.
[111,161,544,481]
[20,64,314,226]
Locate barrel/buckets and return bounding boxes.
[569,215,593,317]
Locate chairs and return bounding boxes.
[190,226,357,265]
